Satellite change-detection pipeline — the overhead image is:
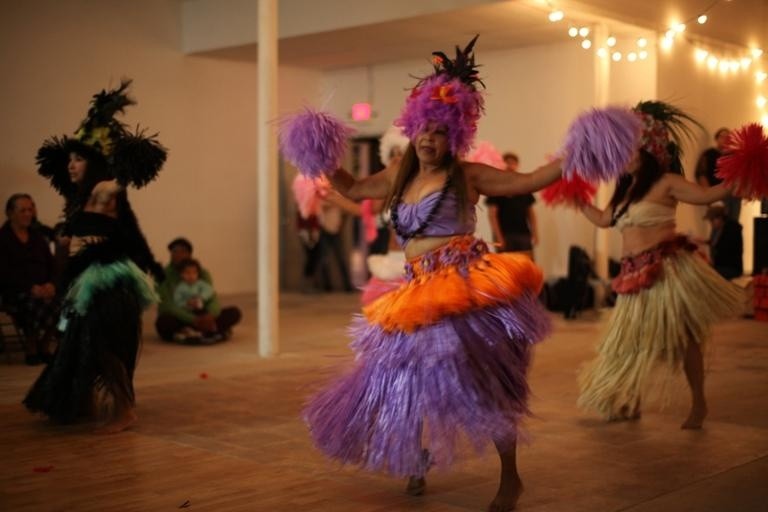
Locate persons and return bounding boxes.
[696,205,744,282]
[0,193,65,366]
[173,258,216,313]
[274,32,645,512]
[364,127,414,280]
[693,126,743,221]
[154,237,241,346]
[539,99,767,432]
[482,152,540,263]
[314,201,354,291]
[17,73,169,436]
